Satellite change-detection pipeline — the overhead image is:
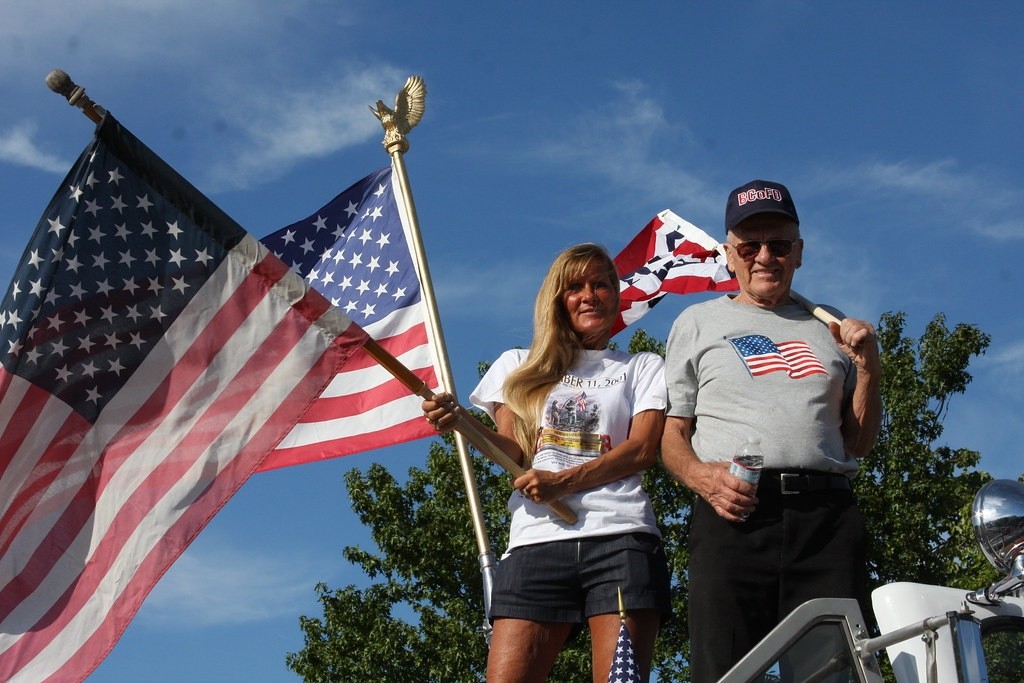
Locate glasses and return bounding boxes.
[728,238,800,260]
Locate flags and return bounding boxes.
[202,72,460,476]
[0,107,371,683]
[587,211,841,338]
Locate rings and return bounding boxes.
[522,487,530,497]
[424,417,432,423]
[435,423,441,430]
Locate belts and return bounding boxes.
[760,472,853,495]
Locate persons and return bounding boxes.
[423,241,664,683]
[660,178,884,682]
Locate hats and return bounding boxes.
[725,180,799,234]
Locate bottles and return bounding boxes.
[728,435,764,517]
